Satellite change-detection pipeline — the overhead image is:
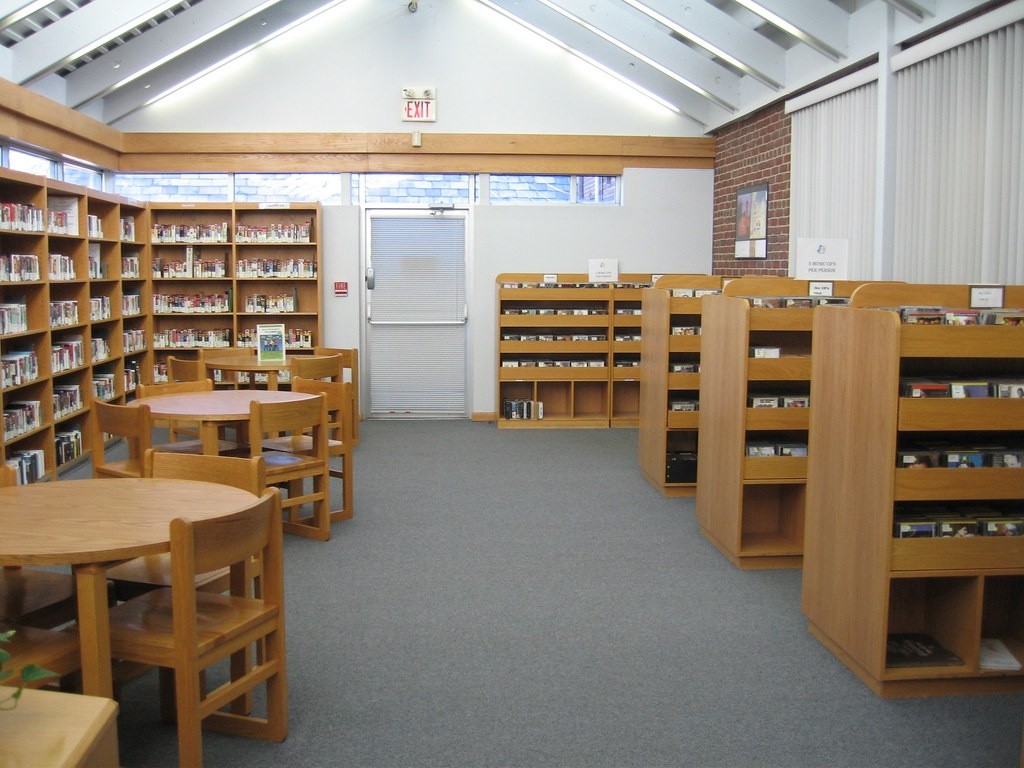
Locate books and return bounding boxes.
[0,200,312,486]
[876,306,1024,674]
[497,275,643,422]
[666,287,850,482]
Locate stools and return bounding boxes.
[0,621,82,695]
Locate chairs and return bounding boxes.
[61,484,289,767]
[0,462,124,702]
[103,446,267,702]
[91,344,361,543]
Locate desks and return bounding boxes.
[125,390,319,456]
[203,354,326,391]
[0,478,261,715]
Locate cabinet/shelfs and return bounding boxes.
[638,274,786,499]
[799,282,1024,699]
[495,272,668,430]
[0,166,325,487]
[693,277,905,573]
